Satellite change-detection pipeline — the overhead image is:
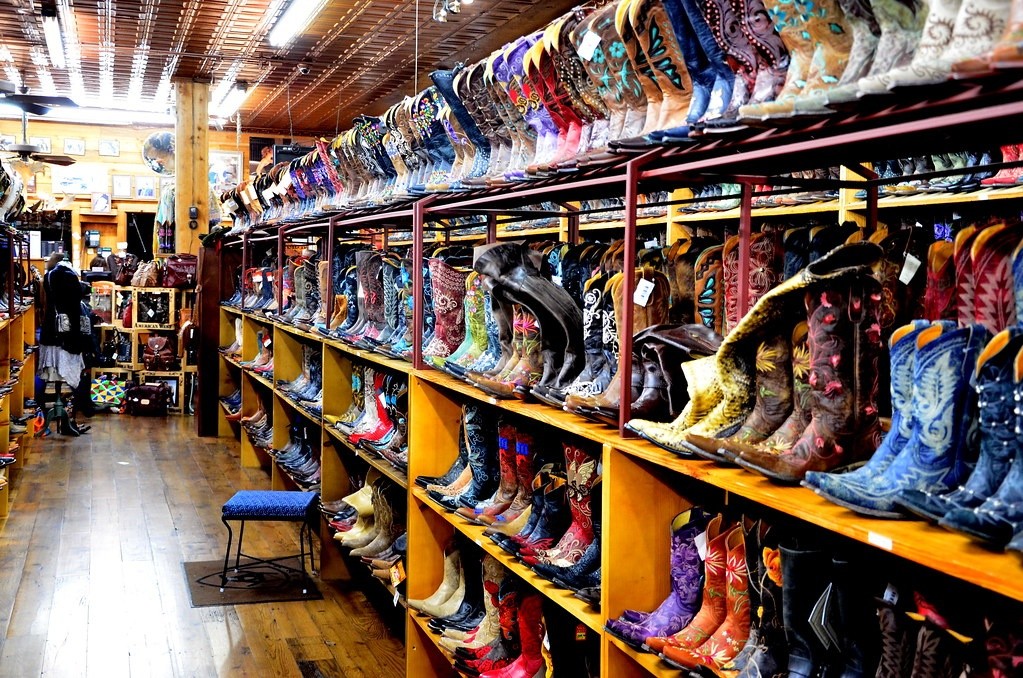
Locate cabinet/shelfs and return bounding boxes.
[80,281,198,413]
[222,59,1023,678]
[0,172,39,532]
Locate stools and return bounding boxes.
[221,489,319,586]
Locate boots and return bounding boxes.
[0,159,37,486]
[220,0,1022,678]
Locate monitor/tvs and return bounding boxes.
[81,269,113,284]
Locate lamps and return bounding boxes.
[432,0,473,23]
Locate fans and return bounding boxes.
[0,73,76,165]
[0,70,79,116]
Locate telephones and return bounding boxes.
[84,230,100,247]
[97,248,112,263]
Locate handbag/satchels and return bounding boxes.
[87,252,197,417]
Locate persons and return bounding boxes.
[35,259,88,389]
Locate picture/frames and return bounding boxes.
[0,134,244,221]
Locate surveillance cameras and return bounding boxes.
[297,62,312,74]
